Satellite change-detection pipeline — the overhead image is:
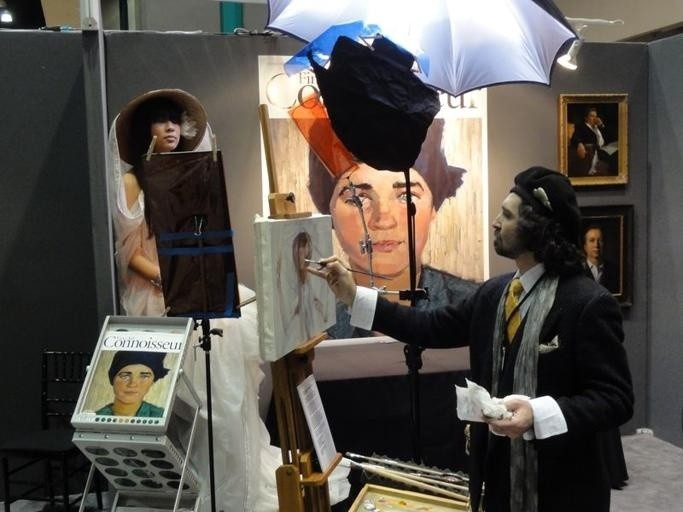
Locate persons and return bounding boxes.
[106,91,305,512]
[570,108,617,175]
[304,119,480,339]
[95,351,165,417]
[310,166,636,510]
[581,224,617,294]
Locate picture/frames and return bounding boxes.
[557,90,631,189]
[575,203,637,311]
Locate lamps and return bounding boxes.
[557,37,584,69]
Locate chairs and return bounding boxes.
[0,346,103,512]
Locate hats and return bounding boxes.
[509,165,583,228]
[108,87,210,168]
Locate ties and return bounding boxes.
[594,265,602,282]
[504,278,522,345]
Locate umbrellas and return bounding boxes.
[266,3,579,95]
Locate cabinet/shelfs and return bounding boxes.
[71,315,205,512]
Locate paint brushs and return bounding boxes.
[305,257,392,283]
